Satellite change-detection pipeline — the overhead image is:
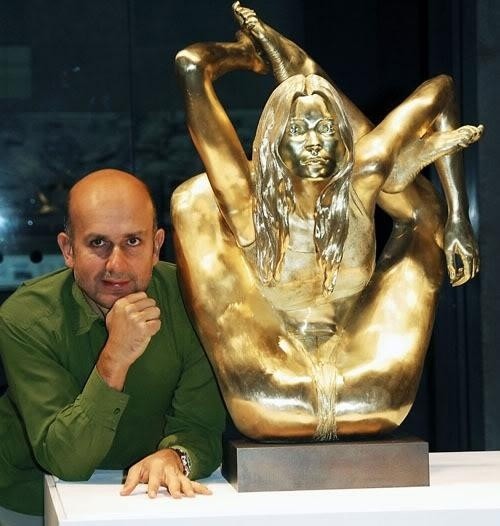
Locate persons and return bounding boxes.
[169,1,484,445]
[0,168,228,526]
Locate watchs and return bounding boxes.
[168,446,192,477]
